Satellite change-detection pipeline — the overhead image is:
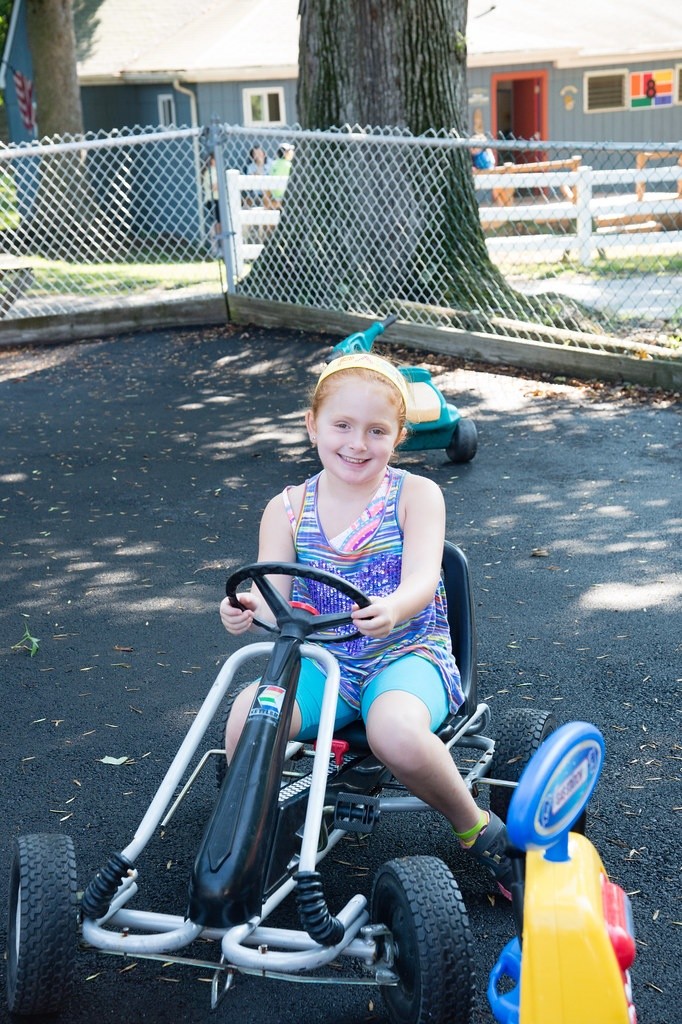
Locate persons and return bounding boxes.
[221,350,524,899]
[201,133,496,235]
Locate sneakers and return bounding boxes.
[460,809,514,902]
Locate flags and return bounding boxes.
[0,0,42,221]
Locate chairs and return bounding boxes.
[310,540,478,750]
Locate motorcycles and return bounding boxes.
[299,315,479,470]
